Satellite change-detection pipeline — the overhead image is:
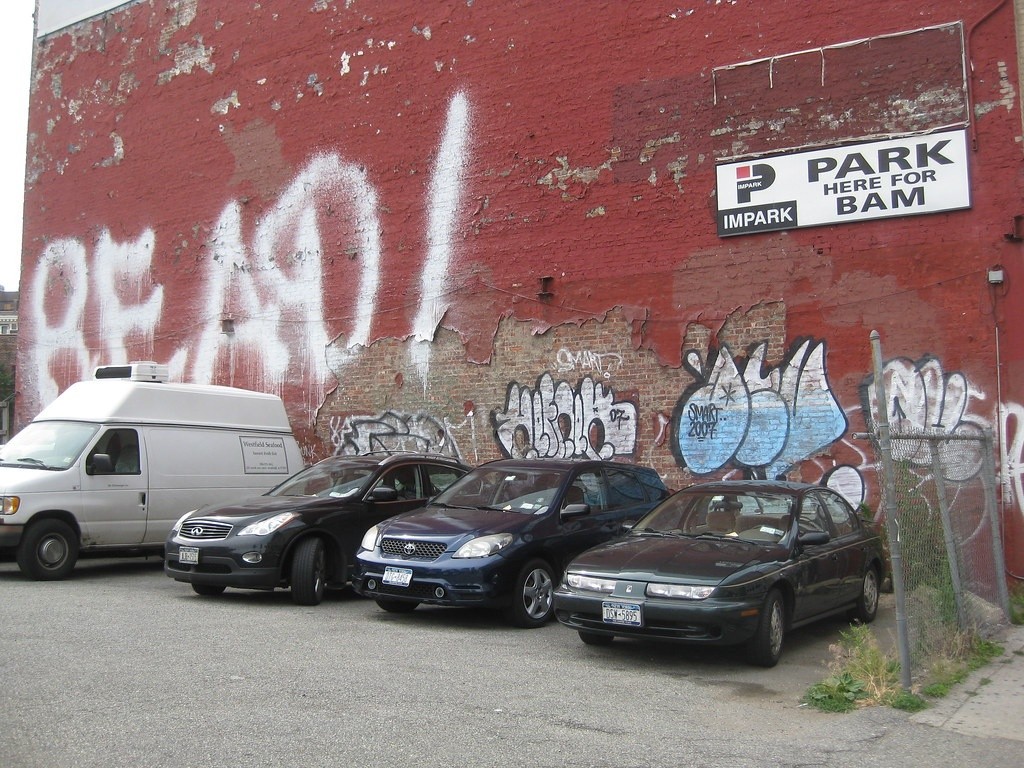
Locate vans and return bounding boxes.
[0,362,308,583]
[357,459,669,629]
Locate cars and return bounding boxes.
[550,481,889,668]
[164,450,475,607]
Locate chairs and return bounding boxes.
[562,486,584,510]
[114,444,139,474]
[706,511,737,535]
[778,515,791,533]
[347,474,367,490]
